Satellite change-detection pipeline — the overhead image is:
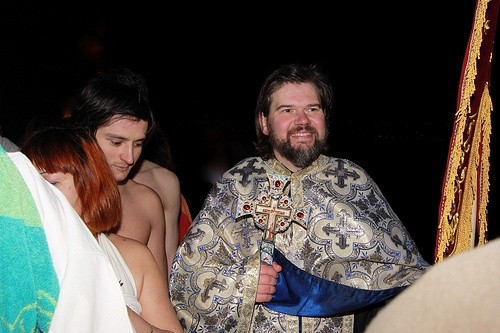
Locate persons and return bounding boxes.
[71,68,182,277]
[19,123,184,333]
[66,74,169,298]
[168,64,432,333]
